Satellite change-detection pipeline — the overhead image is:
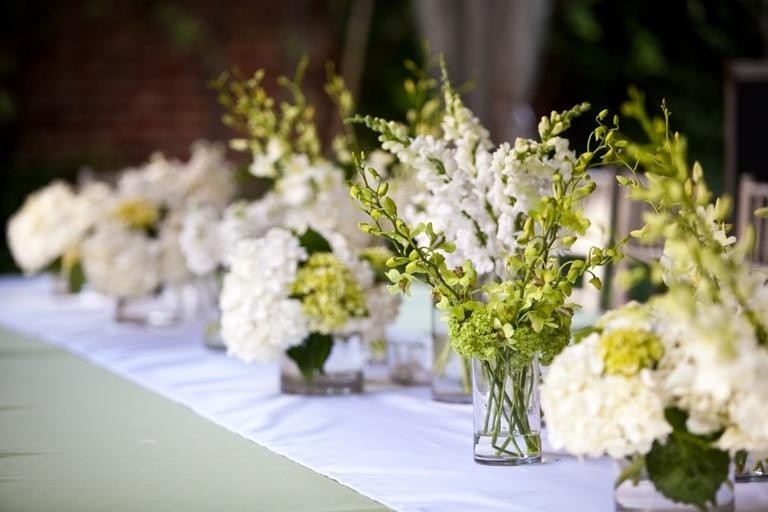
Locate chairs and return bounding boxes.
[732,173,768,266]
[554,170,614,312]
[609,171,683,309]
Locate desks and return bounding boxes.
[0,270,768,509]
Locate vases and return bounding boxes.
[430,299,472,402]
[387,317,431,388]
[614,423,735,510]
[468,341,545,466]
[730,445,768,484]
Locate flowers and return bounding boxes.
[539,288,768,511]
[600,84,768,347]
[334,48,623,457]
[7,49,401,395]
